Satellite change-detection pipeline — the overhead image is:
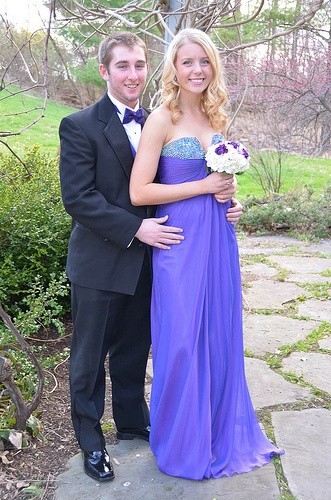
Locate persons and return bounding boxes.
[58,31,245,483]
[129,25,286,482]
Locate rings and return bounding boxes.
[227,180,231,185]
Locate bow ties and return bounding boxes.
[121,108,145,125]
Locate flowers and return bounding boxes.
[204,140,250,175]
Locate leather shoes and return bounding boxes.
[116,424,152,444]
[80,446,116,482]
